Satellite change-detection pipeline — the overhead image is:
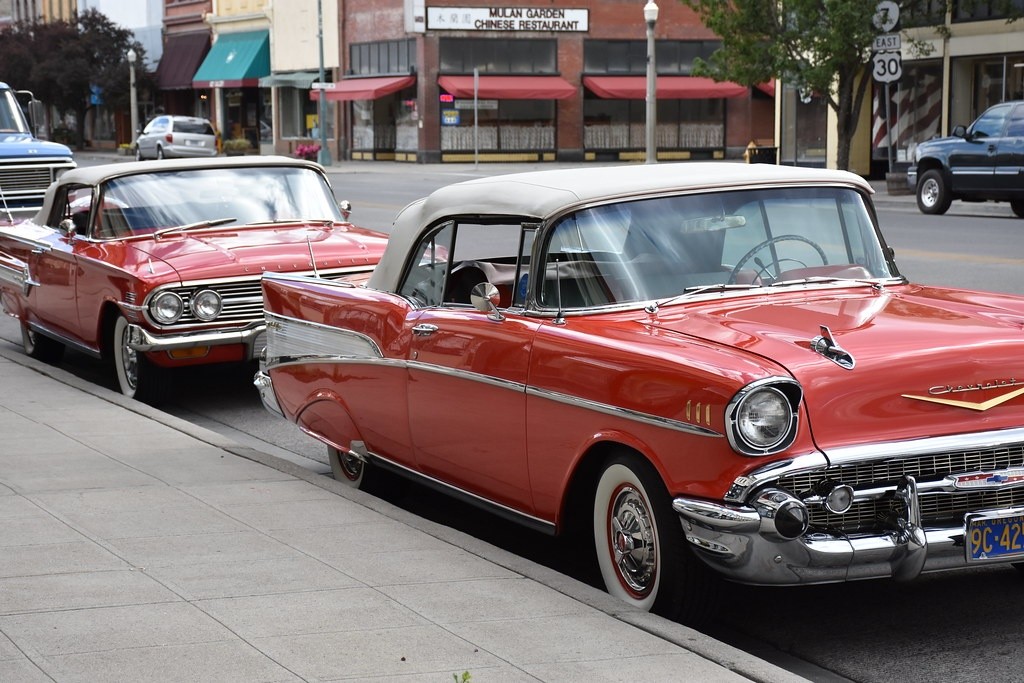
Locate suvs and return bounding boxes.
[135,115,218,162]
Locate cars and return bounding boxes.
[252,160,1024,622]
[0,155,449,407]
[913,101,1023,218]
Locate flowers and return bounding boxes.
[295,144,321,159]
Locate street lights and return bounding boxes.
[127,48,140,147]
[644,1,659,162]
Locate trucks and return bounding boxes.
[0,82,78,219]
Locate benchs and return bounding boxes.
[73,200,254,237]
[447,251,766,309]
